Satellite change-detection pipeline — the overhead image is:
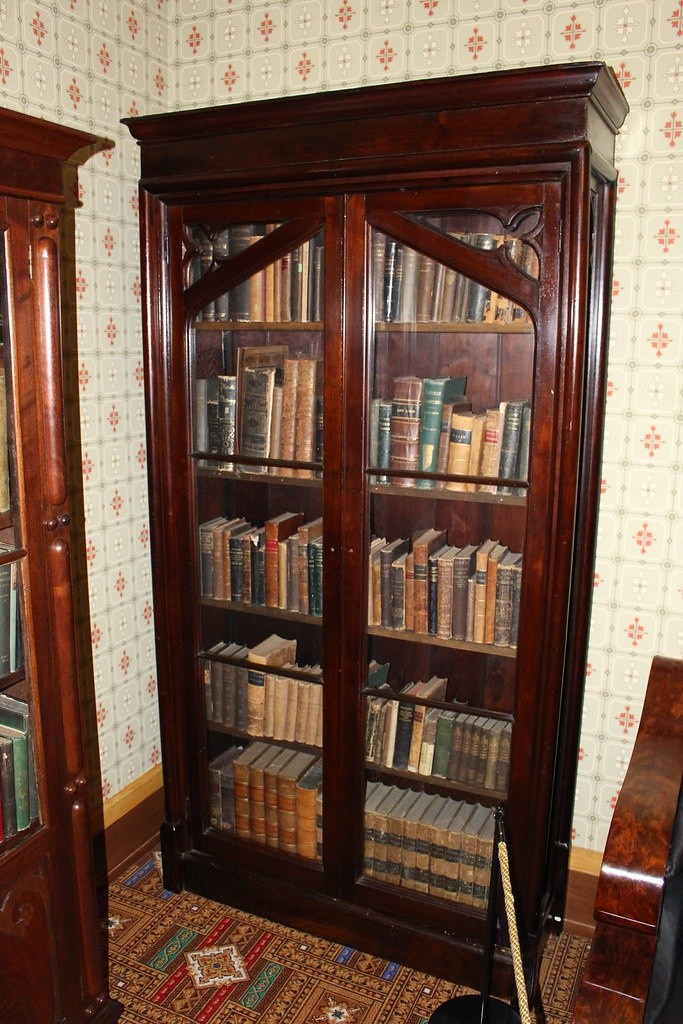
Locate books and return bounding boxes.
[181,197,534,915]
[0,557,46,848]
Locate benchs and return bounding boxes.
[573,657,683,1024]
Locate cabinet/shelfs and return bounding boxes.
[0,102,115,1024]
[120,61,630,1005]
